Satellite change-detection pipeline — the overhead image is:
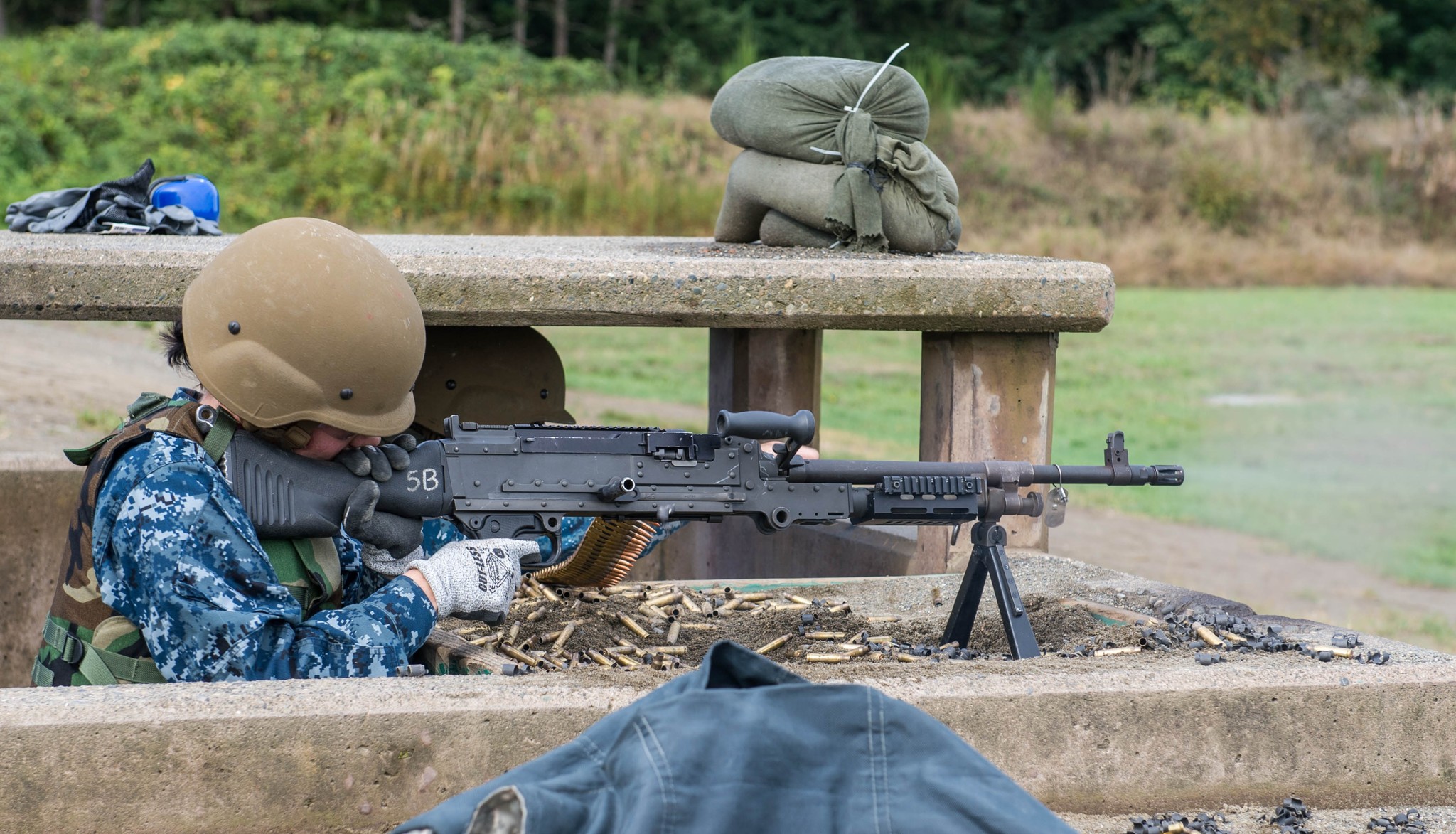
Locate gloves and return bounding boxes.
[333,434,423,575]
[404,537,542,627]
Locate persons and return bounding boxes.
[31,218,819,687]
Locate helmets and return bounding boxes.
[413,326,577,436]
[182,216,427,437]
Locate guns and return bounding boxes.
[193,397,1184,666]
